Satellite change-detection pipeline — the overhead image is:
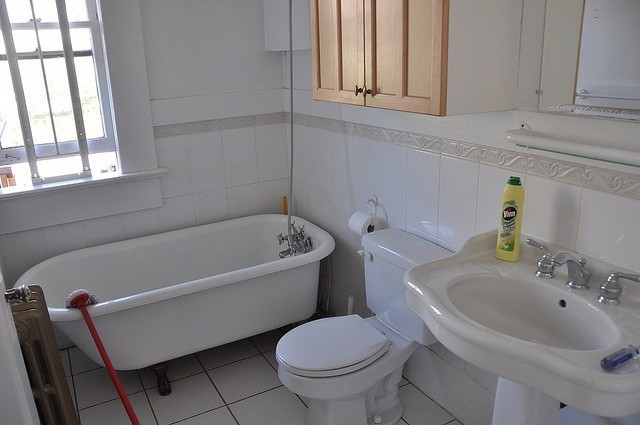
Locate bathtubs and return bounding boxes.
[12,215,335,373]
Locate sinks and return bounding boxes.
[403,229,639,417]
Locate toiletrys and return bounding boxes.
[495,174,525,265]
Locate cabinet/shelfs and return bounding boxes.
[311,1,365,108]
[363,1,448,117]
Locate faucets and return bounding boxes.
[550,252,591,290]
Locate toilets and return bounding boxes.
[276,228,453,423]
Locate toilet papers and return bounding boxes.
[348,210,377,236]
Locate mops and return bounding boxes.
[65,289,141,424]
